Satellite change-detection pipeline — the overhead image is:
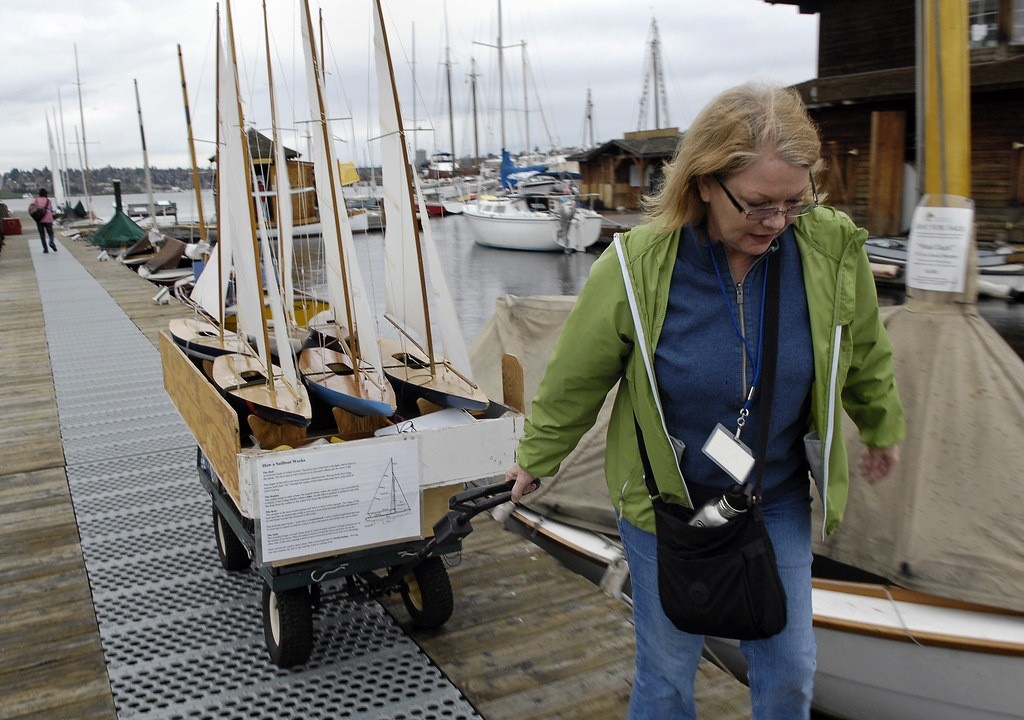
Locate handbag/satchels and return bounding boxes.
[30,207,46,222]
[652,494,792,639]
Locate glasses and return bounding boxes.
[708,173,823,220]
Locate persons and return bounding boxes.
[506,79,907,720]
[29,188,57,253]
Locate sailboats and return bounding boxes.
[471,0,1024,719]
[297,0,398,418]
[309,6,357,351]
[459,1,603,254]
[211,0,313,427]
[376,0,489,411]
[168,2,256,361]
[45,2,554,330]
[243,1,310,357]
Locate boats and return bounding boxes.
[864,236,1024,300]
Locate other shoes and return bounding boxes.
[43,242,57,253]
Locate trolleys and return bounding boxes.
[160,330,541,667]
